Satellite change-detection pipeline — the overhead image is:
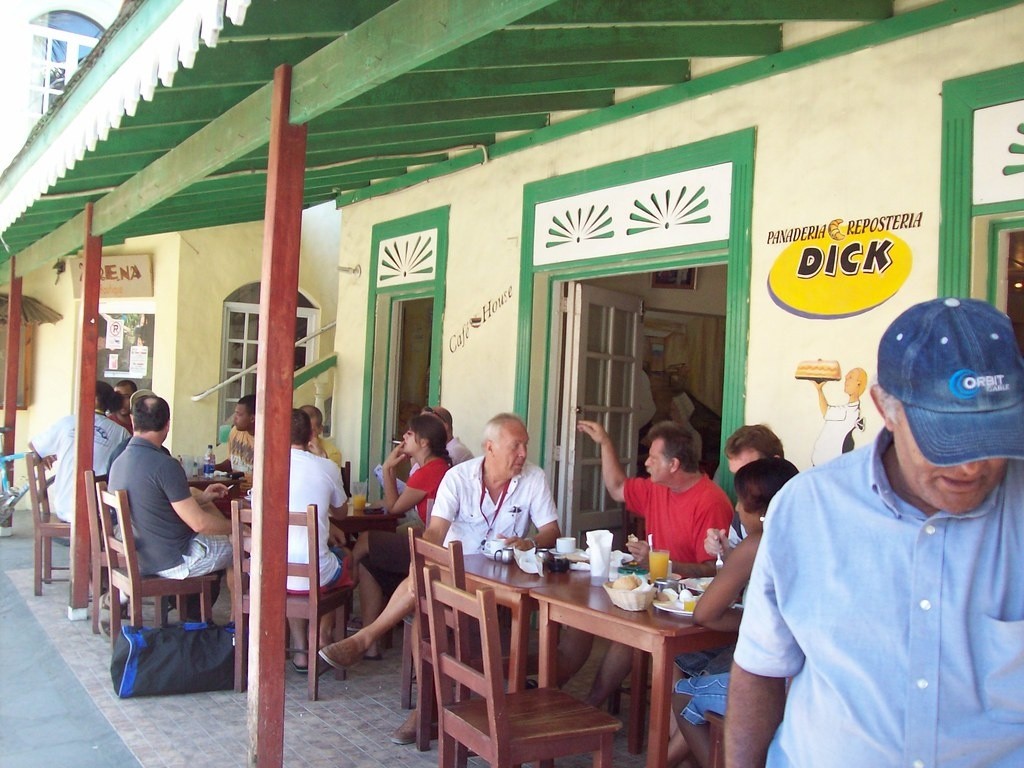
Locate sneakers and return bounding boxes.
[98,594,110,642]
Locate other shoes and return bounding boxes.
[318,638,359,667]
[390,709,438,744]
[347,617,363,631]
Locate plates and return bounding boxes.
[483,551,503,561]
[244,495,251,500]
[679,577,715,593]
[651,596,698,617]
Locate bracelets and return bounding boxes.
[407,455,412,461]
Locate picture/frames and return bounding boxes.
[650,268,698,290]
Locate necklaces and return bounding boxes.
[92,410,108,416]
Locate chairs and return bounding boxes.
[85,468,218,657]
[230,498,352,701]
[399,497,727,768]
[25,451,70,597]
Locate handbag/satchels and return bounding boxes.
[110,620,235,698]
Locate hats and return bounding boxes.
[877,299,1024,466]
[130,388,156,408]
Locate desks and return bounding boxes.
[419,547,628,694]
[213,497,406,534]
[527,573,738,768]
[187,469,248,520]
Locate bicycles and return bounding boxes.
[0,423,58,536]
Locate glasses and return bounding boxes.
[423,406,447,424]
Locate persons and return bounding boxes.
[286,404,474,672]
[724,296,1024,768]
[524,420,735,708]
[28,380,256,643]
[668,424,801,768]
[318,412,561,745]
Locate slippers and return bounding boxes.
[290,656,309,672]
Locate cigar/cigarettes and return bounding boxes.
[392,440,403,444]
[223,484,235,493]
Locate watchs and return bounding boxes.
[524,536,539,550]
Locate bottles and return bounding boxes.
[203,445,215,479]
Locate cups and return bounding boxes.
[247,488,252,496]
[485,538,506,554]
[648,550,670,585]
[181,454,193,480]
[351,482,367,511]
[556,537,576,554]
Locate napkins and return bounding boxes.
[586,529,614,576]
[513,545,543,577]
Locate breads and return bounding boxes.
[612,575,645,591]
